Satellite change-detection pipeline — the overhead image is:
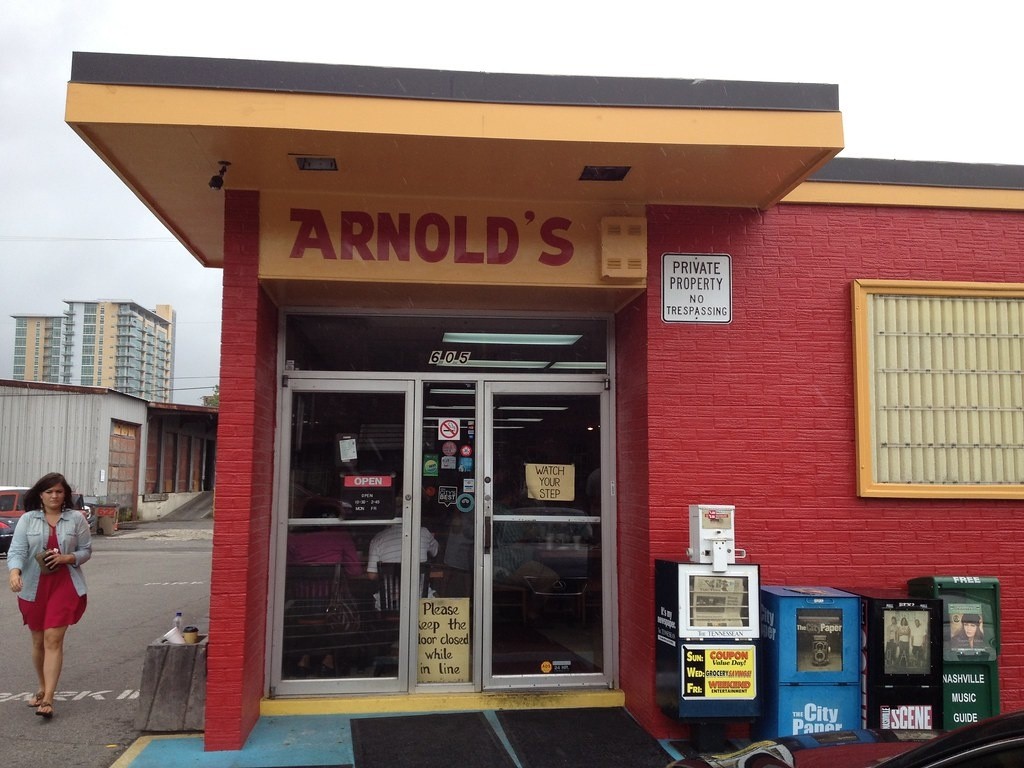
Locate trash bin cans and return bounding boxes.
[96,503,120,535]
[133,633,208,731]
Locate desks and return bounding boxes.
[344,564,444,672]
[525,540,602,622]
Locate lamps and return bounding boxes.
[207,159,229,189]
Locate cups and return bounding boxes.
[164,627,186,645]
[542,533,582,543]
[184,626,200,644]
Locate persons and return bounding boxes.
[440,489,475,599]
[886,616,926,668]
[490,477,562,629]
[951,614,985,649]
[286,495,365,673]
[7,472,93,717]
[366,498,439,611]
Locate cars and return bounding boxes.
[495,504,599,633]
[0,489,87,555]
[667,709,1024,768]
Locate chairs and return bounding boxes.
[281,566,348,676]
[374,559,434,663]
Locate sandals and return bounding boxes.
[35,702,54,718]
[27,689,45,707]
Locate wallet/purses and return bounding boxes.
[35,549,60,575]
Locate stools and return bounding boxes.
[447,567,602,627]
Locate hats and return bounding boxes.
[961,613,979,623]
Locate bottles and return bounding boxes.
[172,612,184,634]
